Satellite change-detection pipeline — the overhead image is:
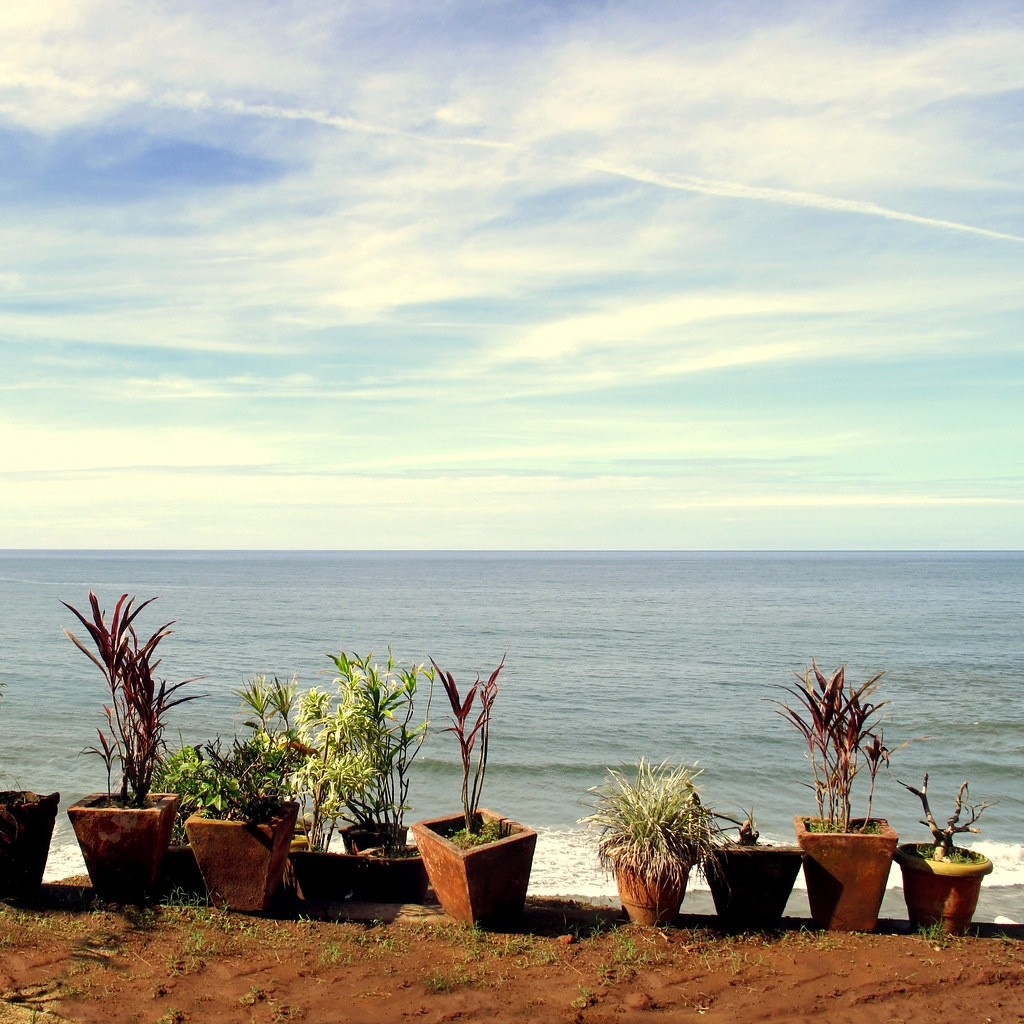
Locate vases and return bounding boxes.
[0,790,61,907]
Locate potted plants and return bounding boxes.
[58,592,994,936]
